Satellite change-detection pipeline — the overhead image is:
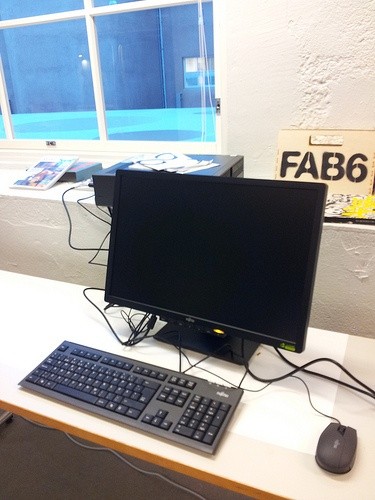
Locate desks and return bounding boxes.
[1,267,375,500]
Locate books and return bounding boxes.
[10,155,78,191]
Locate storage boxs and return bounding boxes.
[57,160,103,183]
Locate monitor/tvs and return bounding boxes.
[104,170,328,365]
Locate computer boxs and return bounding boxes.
[92,152,244,207]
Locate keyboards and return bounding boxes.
[17,340,243,454]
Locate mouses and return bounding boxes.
[316,422,357,474]
